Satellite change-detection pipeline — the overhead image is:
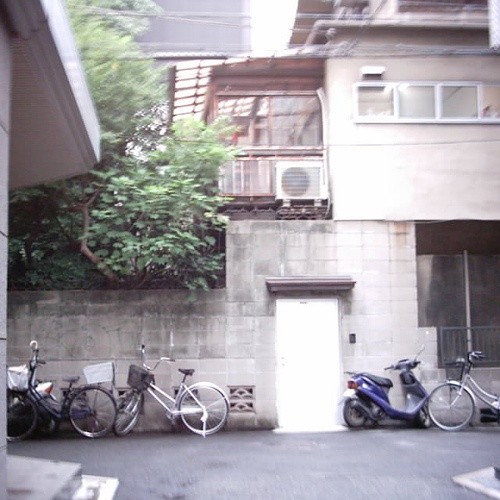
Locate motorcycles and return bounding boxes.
[7,381,58,436]
[337,344,433,430]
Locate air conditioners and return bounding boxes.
[271,157,330,208]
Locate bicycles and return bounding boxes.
[7,340,118,437]
[110,344,230,437]
[426,350,500,432]
